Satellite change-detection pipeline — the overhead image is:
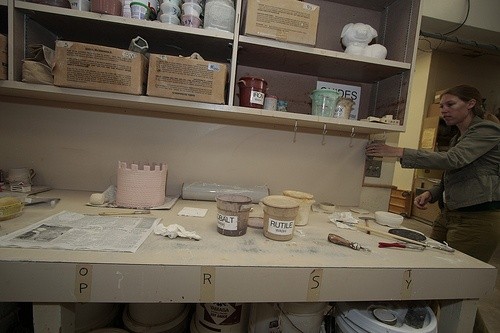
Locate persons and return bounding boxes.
[365,84,500,333]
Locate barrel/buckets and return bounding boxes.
[258,195,300,240]
[281,190,316,226]
[215,194,254,236]
[334,97,356,119]
[237,76,269,109]
[123,303,439,333]
[309,89,340,117]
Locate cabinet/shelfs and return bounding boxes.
[411,117,461,225]
[0,0,426,134]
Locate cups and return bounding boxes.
[129,0,202,28]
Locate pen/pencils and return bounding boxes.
[99,210,151,216]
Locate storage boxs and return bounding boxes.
[240,0,321,46]
[52,39,148,95]
[316,81,362,121]
[429,87,451,117]
[147,52,228,105]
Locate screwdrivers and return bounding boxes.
[327,233,374,254]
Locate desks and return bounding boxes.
[0,182,498,333]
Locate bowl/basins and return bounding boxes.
[375,210,403,228]
[89,0,121,16]
[320,201,336,211]
[0,192,28,221]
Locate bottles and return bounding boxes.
[263,95,277,110]
[204,0,235,33]
